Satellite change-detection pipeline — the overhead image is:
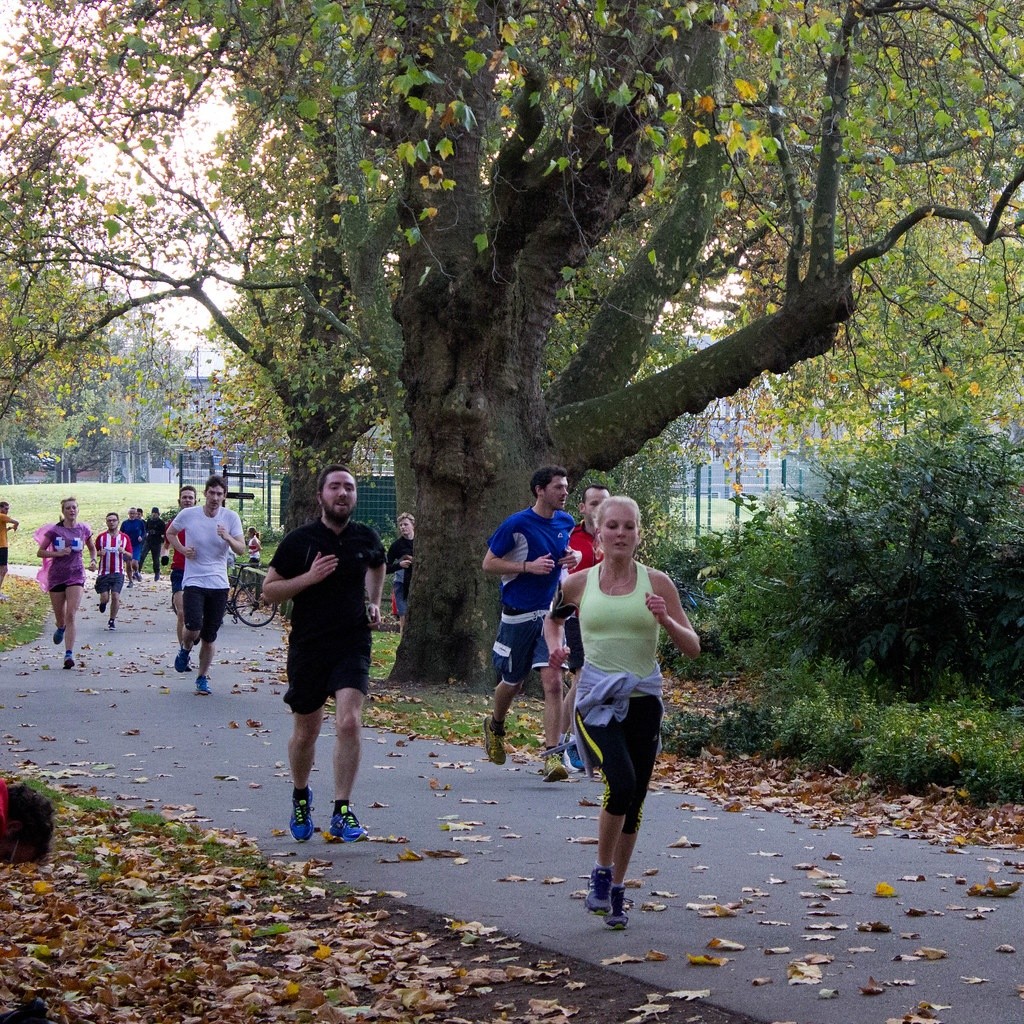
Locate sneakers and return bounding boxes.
[196,675,210,694]
[483,716,507,765]
[585,867,613,915]
[108,618,115,629]
[175,641,193,672]
[563,746,585,772]
[53,625,66,644]
[99,603,106,613]
[62,650,74,669]
[289,787,313,841]
[330,805,368,843]
[601,886,634,930]
[542,753,568,783]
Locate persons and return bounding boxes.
[386,512,415,639]
[138,507,165,581]
[263,465,387,843]
[120,507,146,588]
[163,486,200,672]
[94,513,133,630]
[137,509,146,548]
[0,777,55,866]
[556,485,611,776]
[37,497,97,669]
[0,501,19,600]
[165,476,246,694]
[481,464,583,785]
[247,528,263,569]
[544,496,701,929]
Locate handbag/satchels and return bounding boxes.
[161,545,169,566]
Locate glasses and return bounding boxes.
[107,519,118,522]
[3,839,20,865]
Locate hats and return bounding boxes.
[152,508,160,516]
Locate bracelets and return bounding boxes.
[523,561,525,573]
[91,558,97,564]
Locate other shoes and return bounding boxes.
[133,572,142,583]
[0,594,11,600]
[154,571,160,581]
[127,581,133,588]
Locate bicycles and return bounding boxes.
[172,563,277,627]
